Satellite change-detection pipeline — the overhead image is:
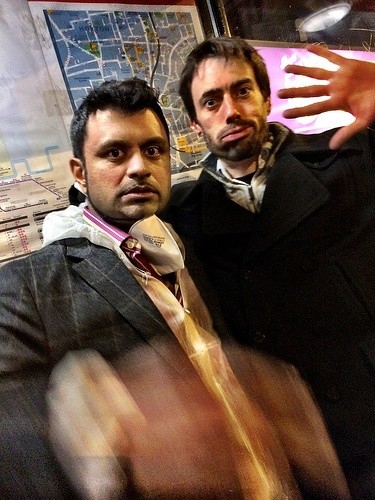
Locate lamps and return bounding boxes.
[297,3,351,33]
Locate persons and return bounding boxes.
[0,75,298,500]
[158,36,375,500]
